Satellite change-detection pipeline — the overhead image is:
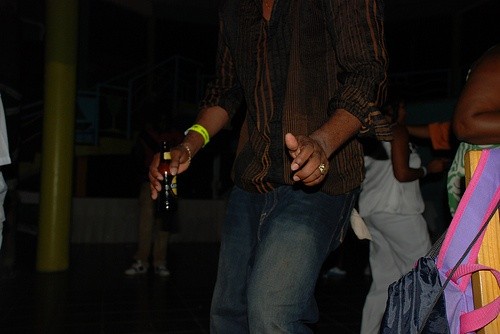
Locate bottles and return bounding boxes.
[158,140,178,214]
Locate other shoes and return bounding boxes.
[126,262,146,275]
[155,267,170,275]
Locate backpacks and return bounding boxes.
[436,146,500,334]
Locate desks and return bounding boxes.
[71,198,224,243]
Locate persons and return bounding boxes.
[400,119,461,159]
[362,90,434,333]
[148,0,392,334]
[126,108,177,279]
[448,42,500,334]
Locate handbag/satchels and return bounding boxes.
[380,257,451,334]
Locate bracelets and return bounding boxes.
[183,123,211,148]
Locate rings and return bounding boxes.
[318,163,327,174]
